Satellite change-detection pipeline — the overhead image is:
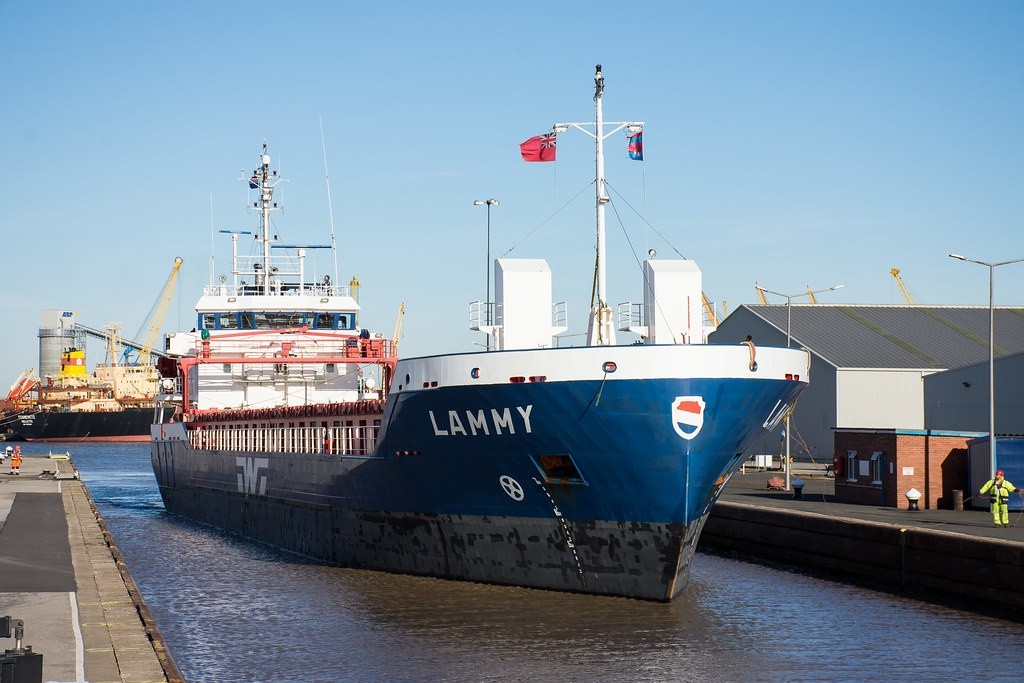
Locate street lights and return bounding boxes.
[754,284,846,491]
[949,253,1024,512]
[473,198,499,352]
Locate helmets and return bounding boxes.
[996,469,1004,477]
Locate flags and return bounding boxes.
[519,132,556,163]
[625,132,643,160]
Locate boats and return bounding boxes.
[148,114,813,606]
[3,320,183,442]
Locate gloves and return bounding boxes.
[975,492,982,500]
[1015,488,1020,492]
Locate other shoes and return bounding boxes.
[993,524,1000,528]
[1004,524,1007,528]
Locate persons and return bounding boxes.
[10,445,22,475]
[978,470,1020,528]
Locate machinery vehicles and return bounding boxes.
[393,301,406,346]
[347,276,360,324]
[128,257,184,366]
[890,267,916,303]
